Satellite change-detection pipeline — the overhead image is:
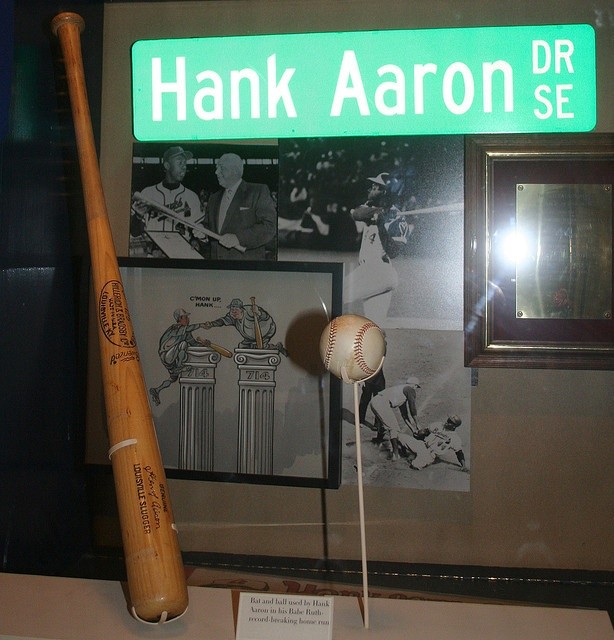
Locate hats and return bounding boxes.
[162,147,194,162]
[407,377,422,389]
[227,299,245,309]
[174,309,191,320]
[447,414,462,428]
[367,174,404,197]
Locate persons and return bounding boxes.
[149,309,211,405]
[354,369,385,430]
[207,299,290,357]
[387,414,470,473]
[344,172,407,318]
[193,153,277,259]
[130,146,206,258]
[370,376,423,462]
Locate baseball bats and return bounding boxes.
[133,191,247,253]
[249,296,263,350]
[196,336,233,358]
[371,202,464,221]
[48,5,191,627]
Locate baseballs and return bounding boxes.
[318,314,385,381]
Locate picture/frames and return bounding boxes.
[76,256,343,490]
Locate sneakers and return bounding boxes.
[278,342,289,358]
[177,366,192,374]
[150,388,162,406]
